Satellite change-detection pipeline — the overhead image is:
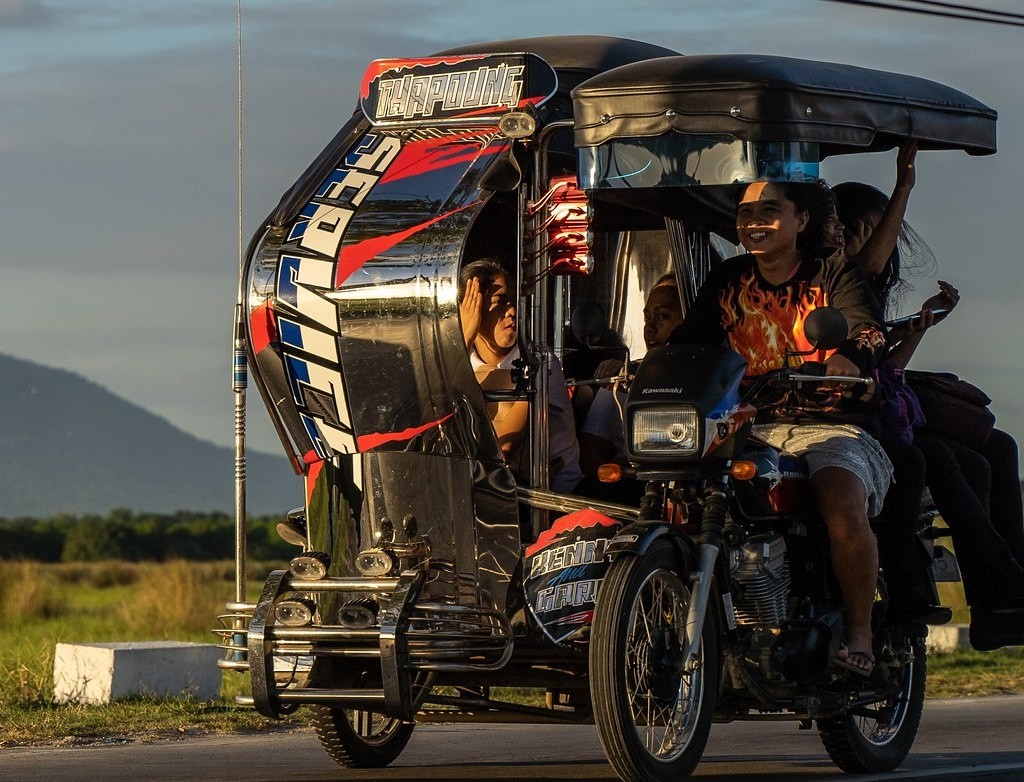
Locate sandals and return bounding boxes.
[833,643,876,676]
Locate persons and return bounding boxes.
[580,273,686,513]
[644,131,1024,674]
[453,259,592,539]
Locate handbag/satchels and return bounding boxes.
[899,371,995,448]
[873,364,926,445]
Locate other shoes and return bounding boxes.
[969,594,1024,651]
[887,601,954,625]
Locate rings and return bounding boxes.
[839,384,848,388]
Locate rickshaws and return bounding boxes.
[208,31,1005,782]
